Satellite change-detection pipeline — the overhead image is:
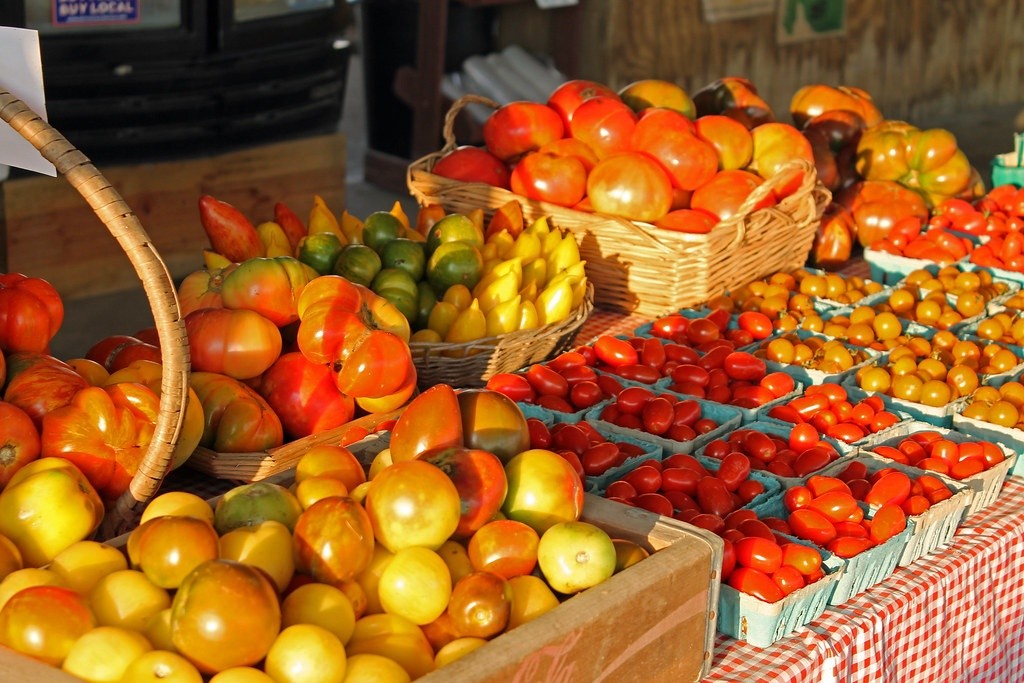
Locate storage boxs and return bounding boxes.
[0,74,1024,683]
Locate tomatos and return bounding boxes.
[0,75,1024,683]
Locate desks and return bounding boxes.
[0,136,349,282]
[704,464,1024,683]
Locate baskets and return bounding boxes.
[188,383,423,481]
[349,237,596,385]
[406,93,839,309]
[477,241,1024,647]
